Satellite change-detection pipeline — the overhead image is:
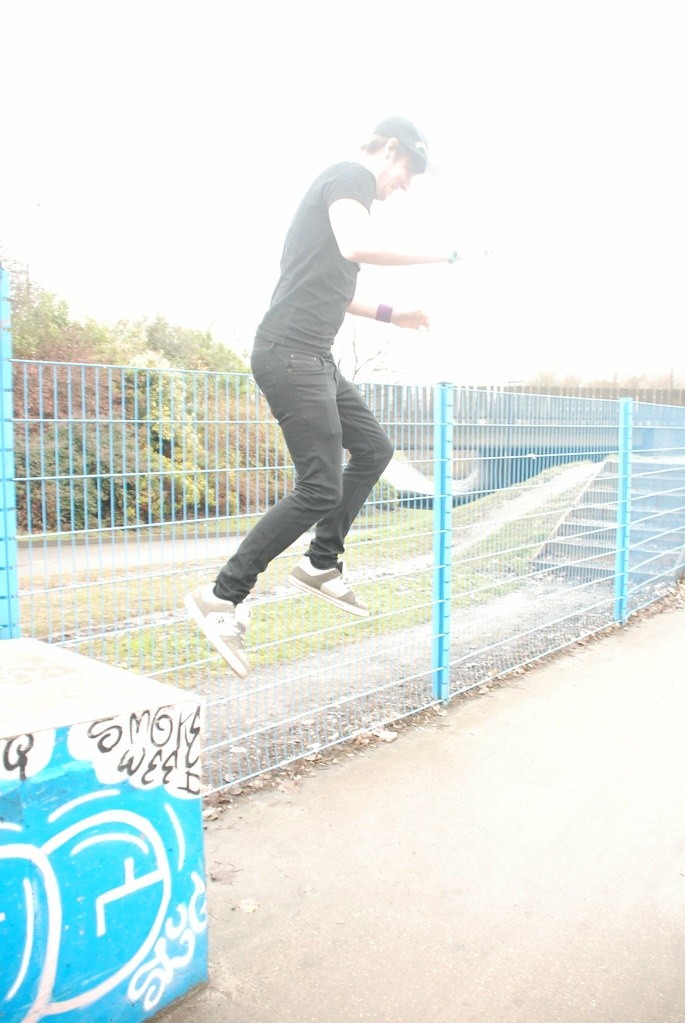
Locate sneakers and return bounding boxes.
[288,556,370,616]
[185,583,247,678]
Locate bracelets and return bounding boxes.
[448,249,458,264]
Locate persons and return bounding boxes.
[180,112,466,682]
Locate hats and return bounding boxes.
[372,117,426,176]
